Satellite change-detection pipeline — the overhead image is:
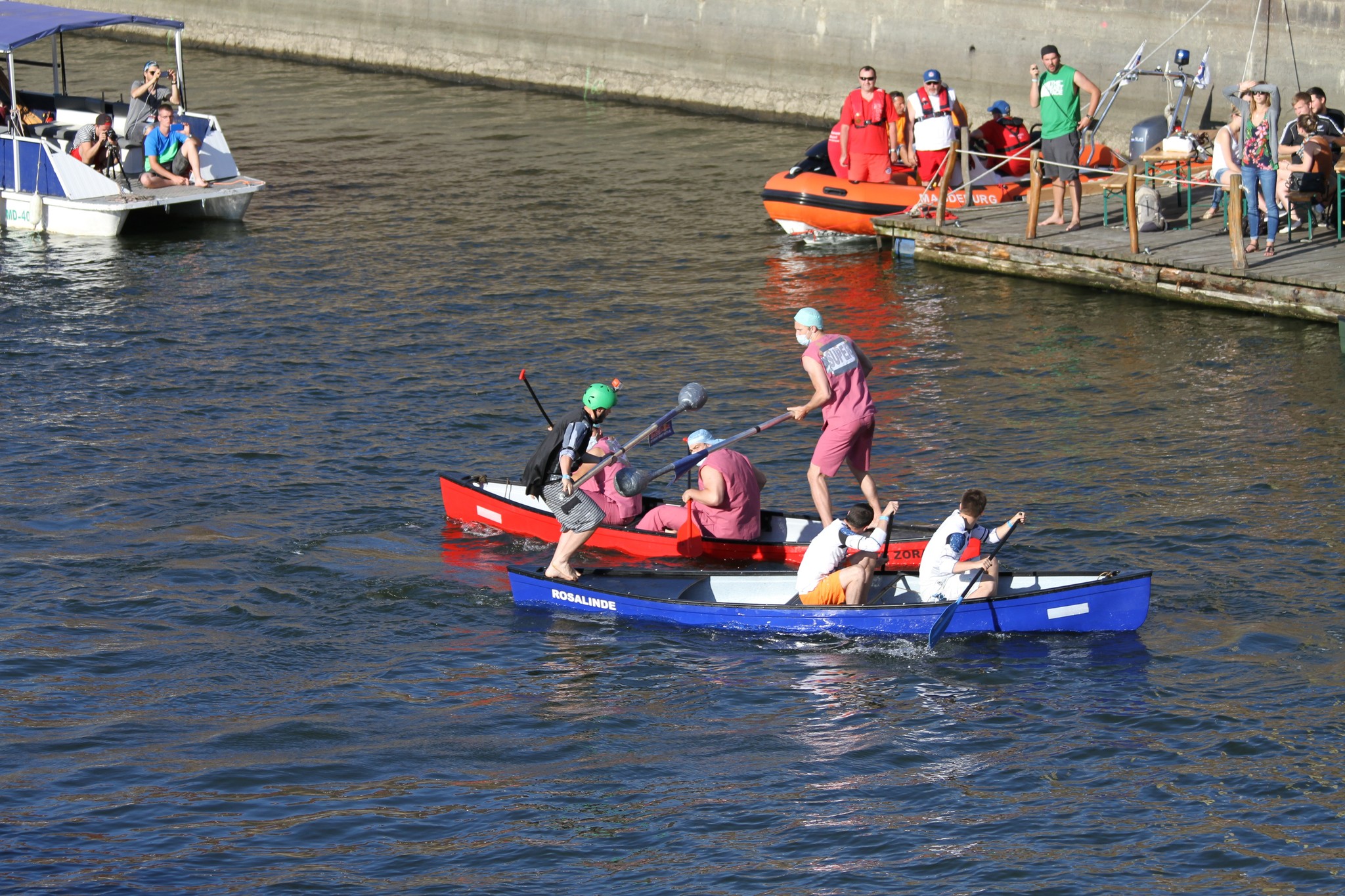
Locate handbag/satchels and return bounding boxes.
[1241,208,1268,237]
[1284,171,1327,193]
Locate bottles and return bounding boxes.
[1175,129,1183,136]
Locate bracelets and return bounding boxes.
[1007,520,1012,528]
[562,475,571,480]
[1286,164,1290,170]
[171,81,177,85]
[187,134,192,138]
[1032,79,1038,82]
[879,516,889,524]
[890,149,897,152]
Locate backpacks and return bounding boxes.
[1326,194,1345,231]
[1110,184,1168,233]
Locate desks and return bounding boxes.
[1139,128,1220,231]
[1334,148,1345,243]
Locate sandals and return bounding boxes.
[1244,244,1260,254]
[1263,246,1274,257]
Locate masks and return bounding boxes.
[691,451,707,467]
[794,307,823,329]
[796,335,810,347]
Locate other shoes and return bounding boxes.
[1279,218,1302,234]
[1277,201,1283,209]
[1264,209,1288,222]
[1203,205,1219,219]
[1311,205,1325,223]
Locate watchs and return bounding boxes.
[1086,114,1094,121]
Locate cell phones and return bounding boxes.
[160,72,169,77]
[170,123,184,132]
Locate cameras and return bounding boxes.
[103,130,118,141]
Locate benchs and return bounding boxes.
[4,113,145,174]
[1100,159,1336,243]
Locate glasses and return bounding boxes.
[1253,91,1265,95]
[926,81,939,85]
[860,76,874,81]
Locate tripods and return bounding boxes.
[105,143,132,192]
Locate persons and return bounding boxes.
[524,307,1025,606]
[828,44,1345,257]
[71,61,209,188]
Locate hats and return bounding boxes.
[987,100,1010,116]
[923,69,941,83]
[1040,44,1058,57]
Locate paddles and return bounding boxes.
[929,516,1021,648]
[677,439,703,555]
[519,369,556,431]
[878,503,894,573]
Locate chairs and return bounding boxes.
[1029,123,1044,149]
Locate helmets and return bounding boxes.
[687,429,726,446]
[582,382,618,409]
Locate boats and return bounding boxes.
[0,0,267,237]
[503,560,1153,632]
[437,468,940,571]
[759,40,1215,237]
